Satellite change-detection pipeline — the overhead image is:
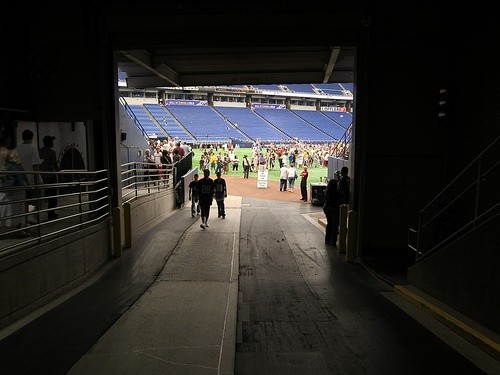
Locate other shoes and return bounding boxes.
[284,189,287,191]
[48,214,59,221]
[17,230,31,238]
[200,224,205,230]
[197,212,200,215]
[218,214,225,219]
[300,198,304,200]
[304,199,307,202]
[280,187,282,191]
[192,213,195,218]
[205,221,210,227]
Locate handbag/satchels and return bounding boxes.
[0,150,18,193]
[250,166,254,172]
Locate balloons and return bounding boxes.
[259,157,266,165]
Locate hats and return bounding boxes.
[43,135,56,144]
[334,170,341,177]
[203,169,210,173]
[243,154,248,158]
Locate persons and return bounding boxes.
[195,169,214,228]
[144,136,192,163]
[13,130,41,218]
[322,179,339,247]
[253,140,332,170]
[288,163,296,193]
[143,149,157,188]
[337,166,351,206]
[279,163,289,192]
[160,149,172,188]
[40,134,60,220]
[301,166,308,202]
[198,142,241,176]
[242,154,250,179]
[189,173,200,218]
[214,172,227,219]
